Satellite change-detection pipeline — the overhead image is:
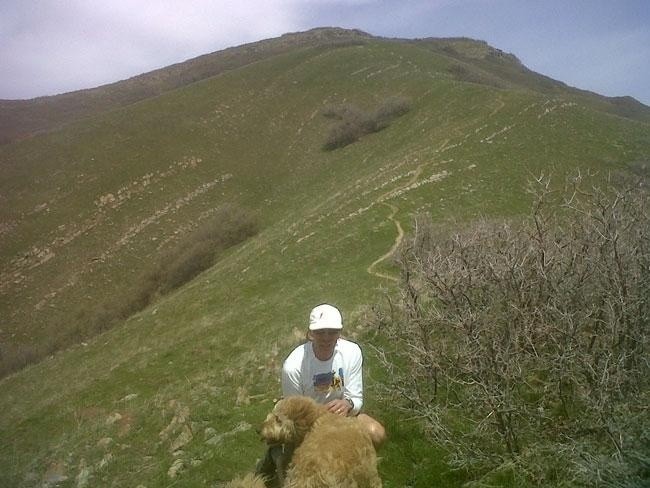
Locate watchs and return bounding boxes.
[348,398,355,410]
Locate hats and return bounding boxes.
[309,304,344,331]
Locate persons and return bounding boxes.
[268,305,385,466]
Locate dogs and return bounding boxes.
[227,395,384,488]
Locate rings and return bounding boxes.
[337,408,342,412]
